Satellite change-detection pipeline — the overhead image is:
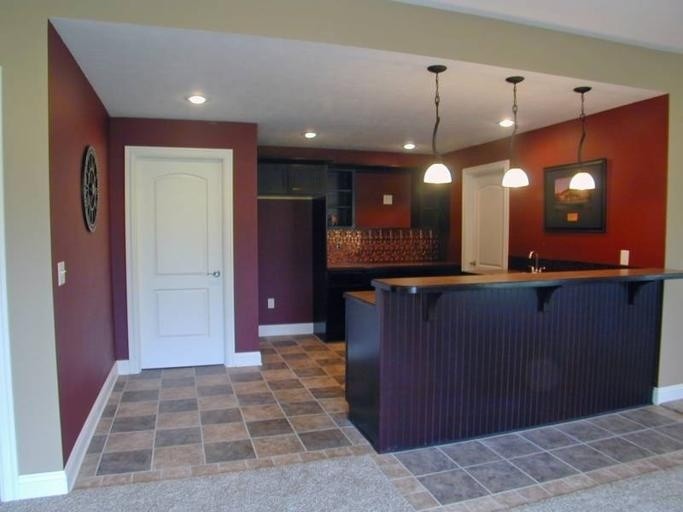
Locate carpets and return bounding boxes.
[1,453,682,512]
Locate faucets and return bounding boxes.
[528,251,547,273]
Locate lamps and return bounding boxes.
[422,64,596,191]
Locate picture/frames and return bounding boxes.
[543,158,607,232]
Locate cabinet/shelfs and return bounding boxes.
[257,162,450,343]
